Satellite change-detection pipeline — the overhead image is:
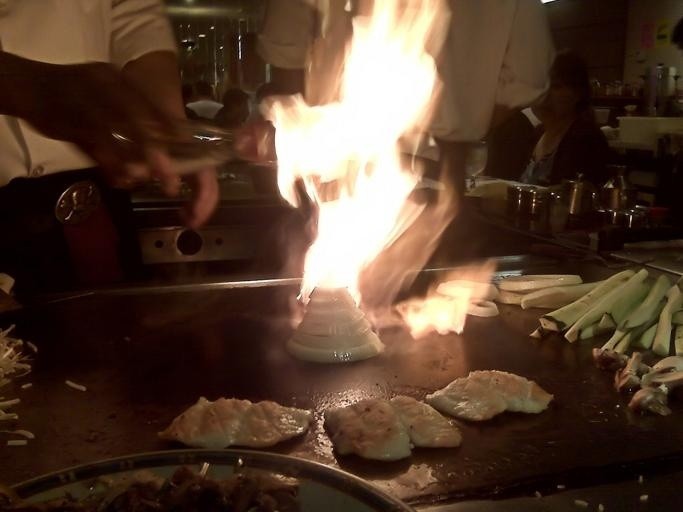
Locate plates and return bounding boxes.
[0,449,416,512]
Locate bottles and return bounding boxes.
[504,168,670,235]
[585,63,683,126]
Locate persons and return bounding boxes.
[255,81,283,121]
[506,54,616,185]
[254,1,559,282]
[0,0,239,310]
[179,82,199,121]
[211,89,249,128]
